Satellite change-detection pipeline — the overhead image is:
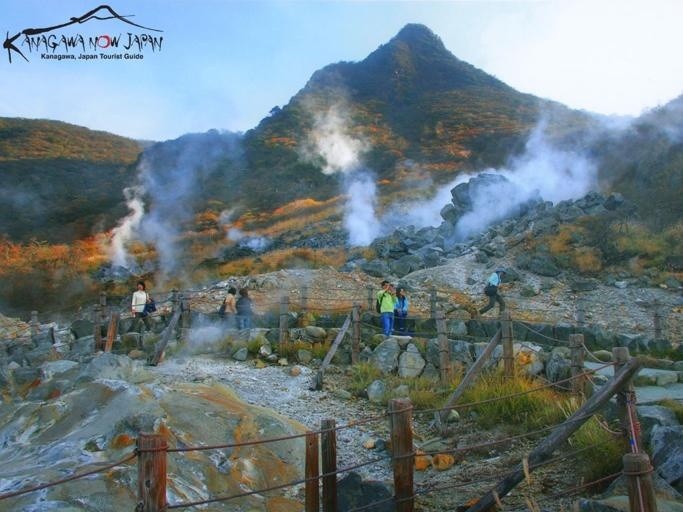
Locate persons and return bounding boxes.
[146,298,156,313]
[223,287,237,329]
[235,289,252,330]
[479,268,506,318]
[375,281,397,337]
[126,281,150,333]
[394,287,409,331]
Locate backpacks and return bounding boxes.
[377,294,384,313]
[219,305,225,318]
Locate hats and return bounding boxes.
[494,267,508,273]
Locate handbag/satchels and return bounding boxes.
[146,300,156,313]
[485,286,497,296]
